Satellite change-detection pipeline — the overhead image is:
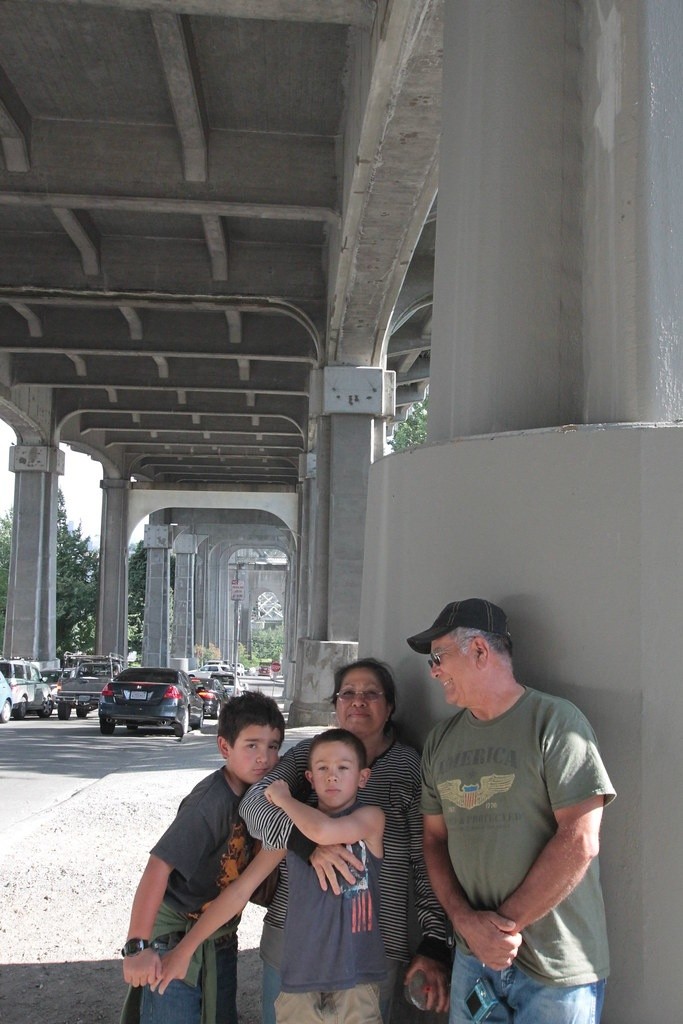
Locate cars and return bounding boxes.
[99,667,204,739]
[186,660,247,720]
[41,668,77,708]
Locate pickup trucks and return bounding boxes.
[56,651,122,720]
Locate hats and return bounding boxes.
[406,598,510,656]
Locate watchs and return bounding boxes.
[122,938,151,957]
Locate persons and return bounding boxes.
[121,691,285,1024]
[236,659,454,1024]
[407,598,617,1024]
[151,729,386,1024]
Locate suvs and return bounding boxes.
[0,655,55,720]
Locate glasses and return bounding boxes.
[336,686,385,702]
[427,635,474,669]
